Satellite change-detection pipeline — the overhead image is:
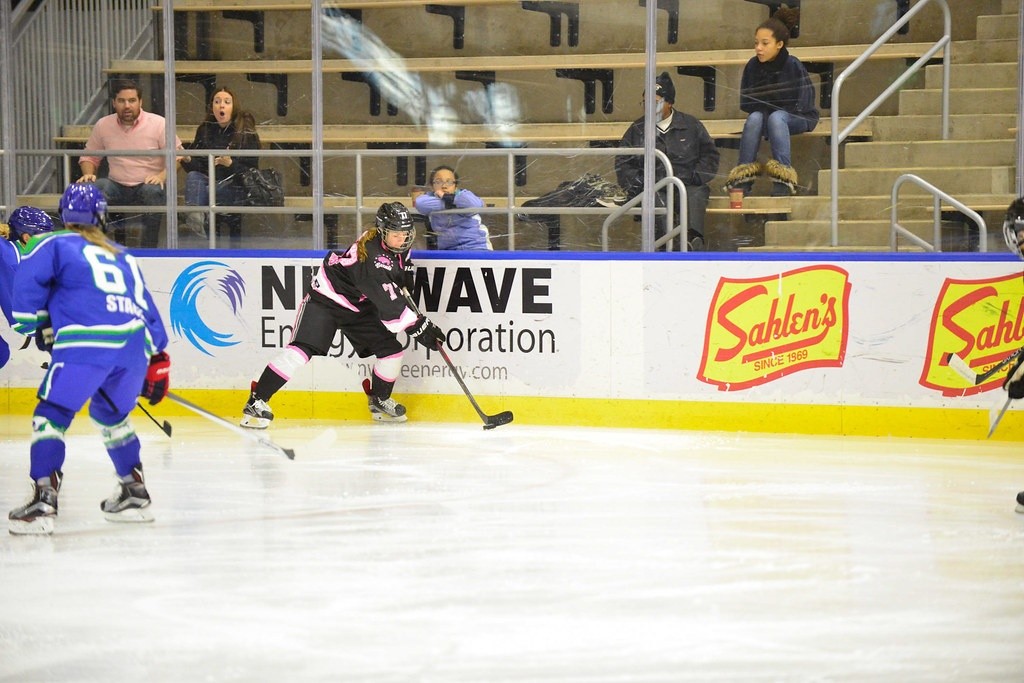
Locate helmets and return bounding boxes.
[58,180,107,233]
[6,204,56,233]
[375,202,418,254]
[1002,197,1024,259]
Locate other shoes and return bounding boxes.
[688,233,705,250]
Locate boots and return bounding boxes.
[188,212,209,240]
[724,163,762,197]
[766,159,796,197]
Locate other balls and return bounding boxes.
[483,425,496,430]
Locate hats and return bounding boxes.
[642,71,677,106]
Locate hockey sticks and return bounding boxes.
[167,391,295,461]
[137,401,172,438]
[401,287,514,427]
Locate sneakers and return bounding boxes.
[361,379,408,423]
[6,472,62,535]
[239,380,274,429]
[99,470,156,522]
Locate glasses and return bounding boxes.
[433,180,457,187]
[641,99,666,106]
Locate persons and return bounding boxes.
[0,205,54,373]
[77,79,184,250]
[1002,197,1023,515]
[9,184,168,535]
[241,201,446,430]
[615,71,720,252]
[176,88,262,236]
[418,166,493,251]
[724,19,819,196]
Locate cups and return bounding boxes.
[411,188,425,207]
[729,188,743,208]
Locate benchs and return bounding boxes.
[14,0,1022,251]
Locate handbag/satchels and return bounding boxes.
[219,168,287,215]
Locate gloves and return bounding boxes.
[400,260,415,293]
[1002,348,1024,400]
[141,349,171,406]
[35,325,55,352]
[406,316,447,352]
